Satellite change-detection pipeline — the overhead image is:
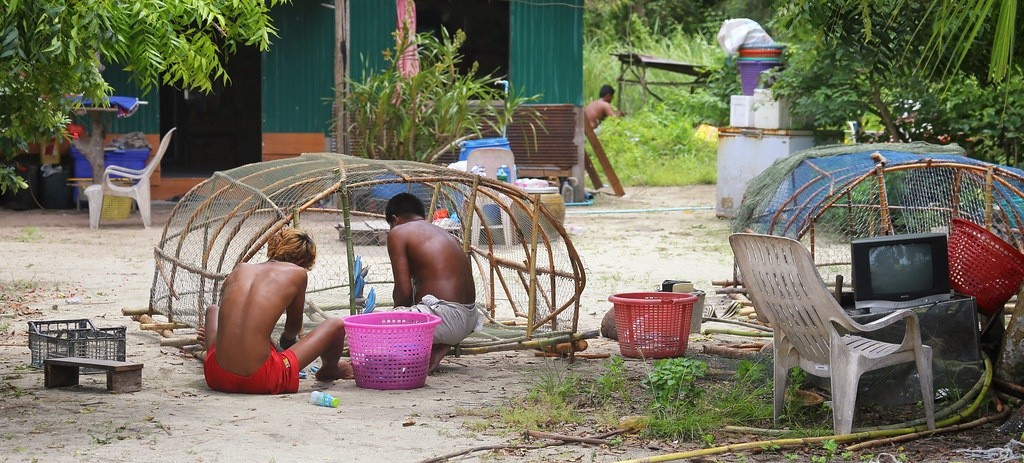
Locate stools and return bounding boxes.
[44,357,144,394]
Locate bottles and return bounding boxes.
[501,164,511,183]
[561,182,573,203]
[496,166,508,183]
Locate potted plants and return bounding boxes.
[318,19,551,213]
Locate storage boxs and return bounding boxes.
[70,146,150,201]
[730,95,754,128]
[25,318,128,370]
[753,89,815,130]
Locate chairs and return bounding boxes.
[729,232,935,436]
[462,146,517,248]
[84,127,177,230]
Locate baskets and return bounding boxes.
[608,291,699,358]
[736,45,787,96]
[948,217,1024,318]
[26,318,127,374]
[343,311,442,389]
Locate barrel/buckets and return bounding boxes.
[665,291,706,334]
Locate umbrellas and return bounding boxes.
[397,1,418,107]
[43,95,150,118]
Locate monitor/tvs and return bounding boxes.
[850,232,950,313]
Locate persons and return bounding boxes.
[584,85,625,130]
[384,193,478,376]
[206,229,355,393]
